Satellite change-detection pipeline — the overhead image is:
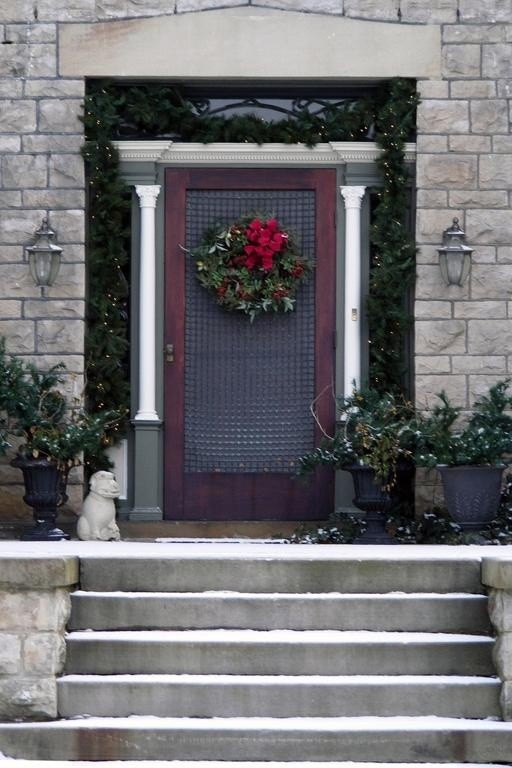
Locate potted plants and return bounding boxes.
[285,378,429,543]
[414,378,512,530]
[0,337,129,542]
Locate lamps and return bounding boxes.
[436,217,474,287]
[26,218,64,286]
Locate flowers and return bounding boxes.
[192,208,318,325]
